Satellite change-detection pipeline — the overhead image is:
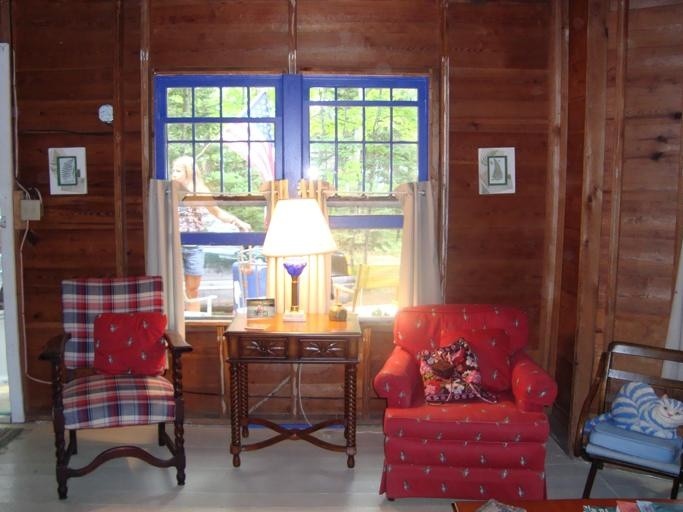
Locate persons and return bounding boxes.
[170,155,255,311]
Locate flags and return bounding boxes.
[221,91,275,225]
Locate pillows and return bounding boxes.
[92,311,168,378]
[417,337,483,403]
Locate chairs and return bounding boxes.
[573,341,683,499]
[374,305,559,502]
[182,265,217,317]
[38,276,195,499]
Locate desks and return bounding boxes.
[224,313,364,468]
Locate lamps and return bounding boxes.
[261,198,339,323]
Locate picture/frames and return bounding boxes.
[478,147,516,196]
[48,147,88,196]
